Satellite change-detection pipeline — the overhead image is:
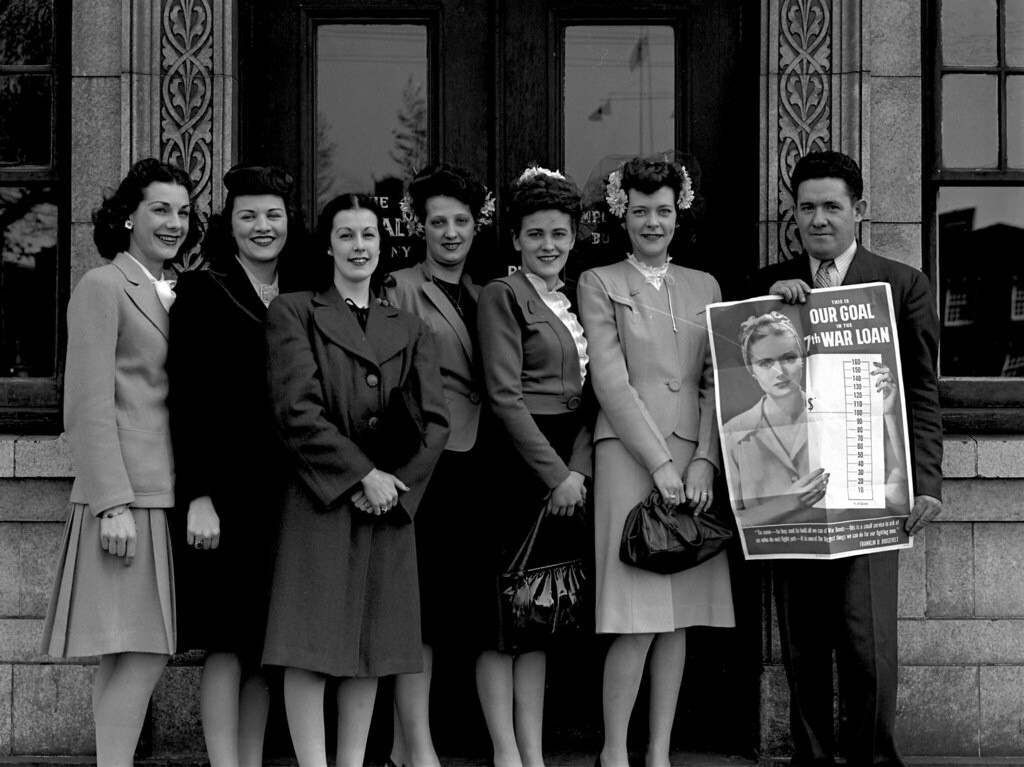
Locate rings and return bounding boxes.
[381,508,387,513]
[669,495,676,499]
[195,541,203,549]
[701,492,708,495]
[889,378,894,383]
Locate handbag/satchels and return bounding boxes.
[620,488,732,573]
[498,494,604,654]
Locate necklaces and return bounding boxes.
[433,278,464,316]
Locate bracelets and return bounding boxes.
[102,506,128,519]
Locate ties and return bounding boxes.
[814,258,834,289]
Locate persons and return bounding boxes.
[574,157,735,767]
[262,193,449,767]
[459,166,598,767]
[37,157,205,767]
[166,165,320,767]
[720,311,908,527]
[737,151,947,767]
[379,161,494,767]
